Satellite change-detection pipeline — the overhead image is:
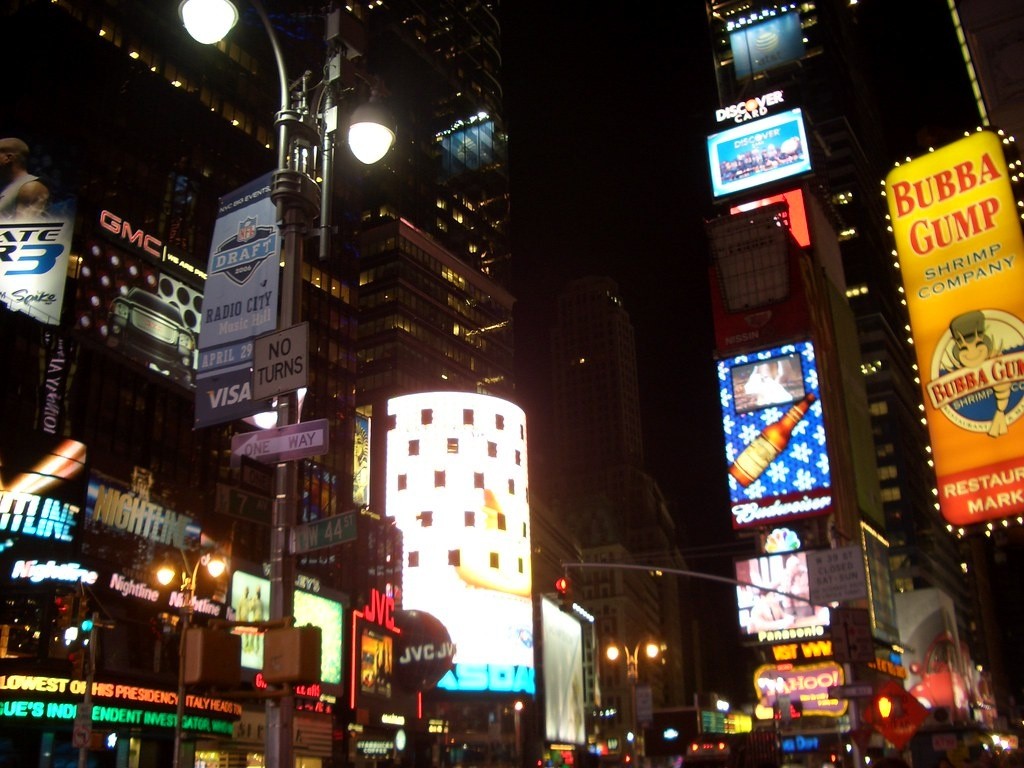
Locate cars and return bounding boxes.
[684,731,735,768]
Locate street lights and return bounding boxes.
[154,535,231,768]
[601,633,664,768]
[178,1,398,767]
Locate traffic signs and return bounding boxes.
[827,684,875,700]
[231,420,328,472]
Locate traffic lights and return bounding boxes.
[555,576,569,595]
